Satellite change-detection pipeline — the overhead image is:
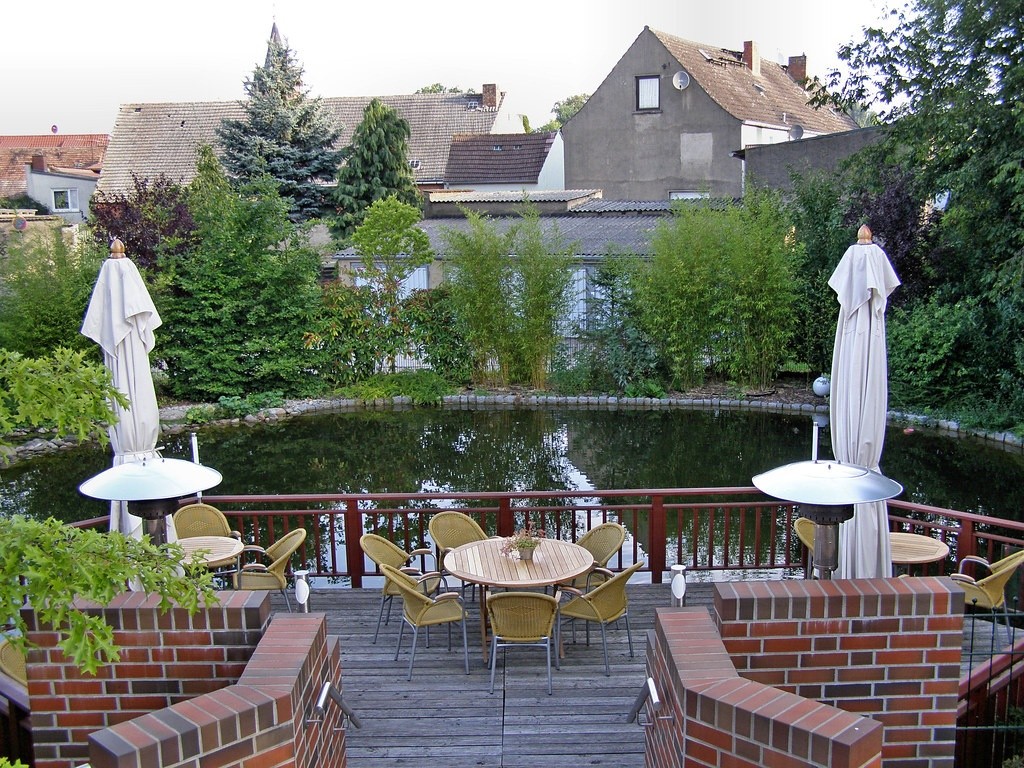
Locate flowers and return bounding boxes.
[499,518,543,558]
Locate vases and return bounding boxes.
[518,547,535,560]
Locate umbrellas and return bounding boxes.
[80,236,186,593]
[827,227,902,580]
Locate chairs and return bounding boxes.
[173,504,243,596]
[547,523,625,642]
[484,593,558,696]
[234,528,306,613]
[378,564,470,682]
[950,550,1024,651]
[559,561,644,679]
[0,636,28,688]
[429,512,507,600]
[360,535,440,648]
[794,517,817,580]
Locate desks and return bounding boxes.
[889,532,950,578]
[443,538,594,658]
[168,536,244,596]
